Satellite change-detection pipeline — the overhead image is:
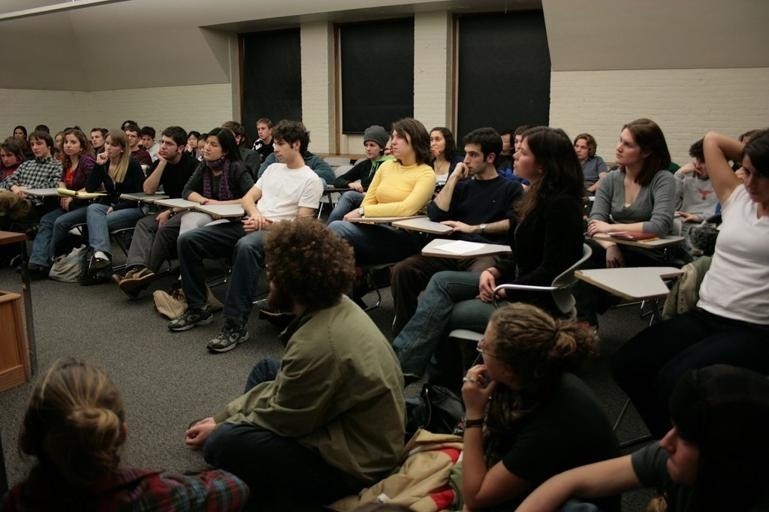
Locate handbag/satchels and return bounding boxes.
[49,243,89,284]
[80,263,126,286]
[153,284,223,320]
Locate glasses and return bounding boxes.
[474,338,497,359]
[159,140,174,146]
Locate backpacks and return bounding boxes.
[33,123,50,134]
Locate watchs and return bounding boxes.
[463,416,486,428]
[356,207,366,219]
[480,224,489,234]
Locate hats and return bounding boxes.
[363,125,388,146]
[670,364,769,465]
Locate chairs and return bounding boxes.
[318,165,352,219]
[120,192,177,272]
[63,191,137,275]
[24,188,86,248]
[596,229,685,250]
[573,263,684,447]
[153,198,200,279]
[196,179,328,298]
[347,216,421,311]
[323,186,351,210]
[390,217,454,331]
[1,188,28,269]
[420,238,591,386]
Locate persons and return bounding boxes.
[325,125,394,227]
[165,118,323,354]
[571,132,609,196]
[26,128,98,281]
[386,124,587,390]
[428,124,461,200]
[0,135,29,181]
[12,125,31,158]
[325,117,436,269]
[388,126,524,334]
[0,131,64,220]
[151,127,257,321]
[90,126,110,159]
[121,119,153,179]
[498,123,534,189]
[182,215,406,511]
[139,125,161,178]
[672,138,724,238]
[186,130,208,161]
[574,116,678,328]
[110,125,204,300]
[257,131,334,187]
[617,125,769,423]
[249,118,277,164]
[221,119,262,186]
[512,363,768,511]
[84,127,149,287]
[457,300,625,512]
[54,130,66,159]
[0,355,251,512]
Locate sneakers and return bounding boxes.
[206,324,249,352]
[258,300,296,316]
[118,266,155,288]
[167,302,214,331]
[112,273,141,298]
